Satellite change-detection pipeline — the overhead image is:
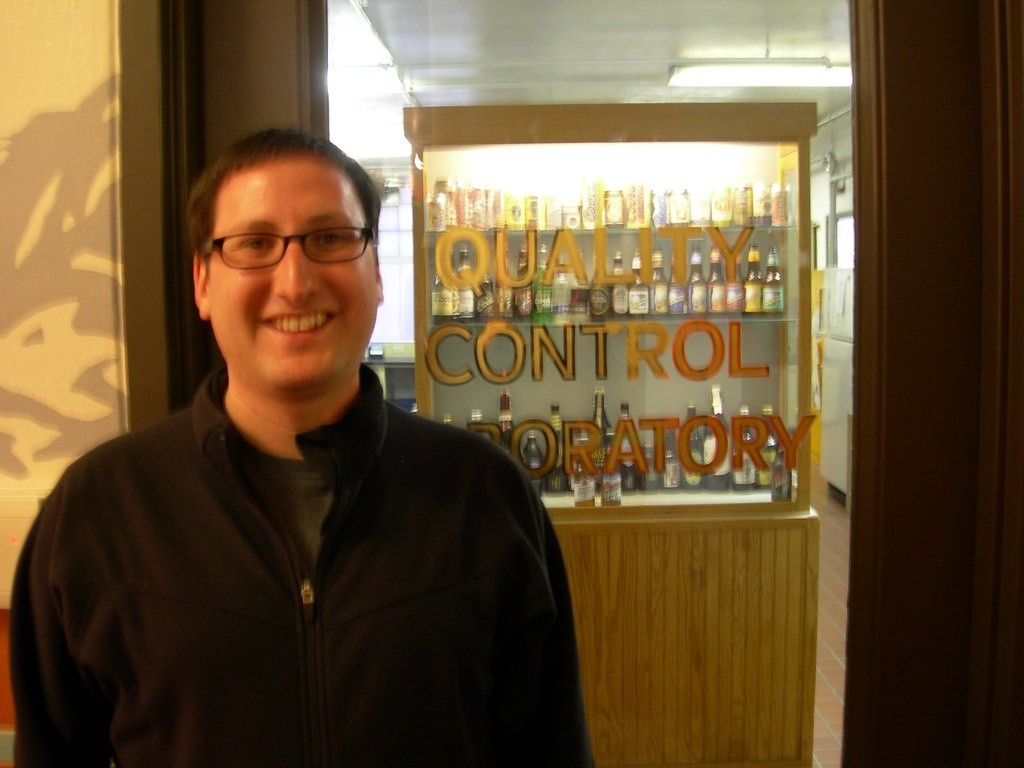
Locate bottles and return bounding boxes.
[515,245,532,315]
[611,251,628,315]
[474,274,494,317]
[756,404,777,489]
[680,405,704,489]
[452,250,473,319]
[668,253,686,314]
[707,247,724,313]
[533,244,551,313]
[744,243,761,314]
[431,274,452,319]
[496,249,514,317]
[731,405,754,490]
[662,429,679,489]
[725,256,741,312]
[442,389,659,508]
[591,257,610,318]
[553,274,569,313]
[649,247,668,313]
[762,247,784,315]
[705,387,730,491]
[627,248,649,314]
[687,246,707,313]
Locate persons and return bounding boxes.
[7,127,594,768]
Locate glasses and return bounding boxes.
[203,226,375,270]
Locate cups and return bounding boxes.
[426,179,788,230]
[570,284,589,314]
[773,450,791,500]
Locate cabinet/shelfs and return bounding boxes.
[403,102,821,768]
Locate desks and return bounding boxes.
[362,342,416,400]
